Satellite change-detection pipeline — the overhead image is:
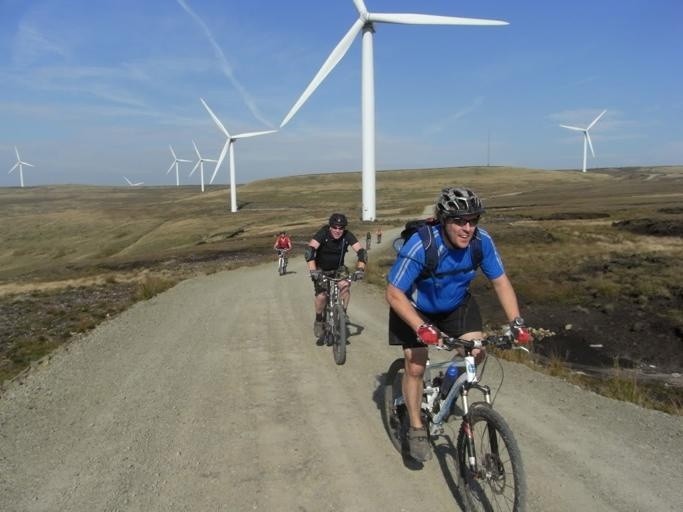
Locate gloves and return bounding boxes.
[352,269,364,281]
[309,270,321,281]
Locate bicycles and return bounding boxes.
[276,248,288,274]
[311,272,352,365]
[384,333,527,512]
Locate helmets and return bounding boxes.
[329,213,347,227]
[436,187,485,227]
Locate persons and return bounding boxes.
[304,212,367,339]
[272,229,292,271]
[385,186,532,463]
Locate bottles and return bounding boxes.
[440,365,461,396]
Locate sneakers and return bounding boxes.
[407,424,433,461]
[452,402,465,416]
[314,320,324,337]
[345,314,349,324]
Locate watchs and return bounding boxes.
[509,317,524,326]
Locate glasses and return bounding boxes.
[331,226,344,230]
[447,217,480,226]
